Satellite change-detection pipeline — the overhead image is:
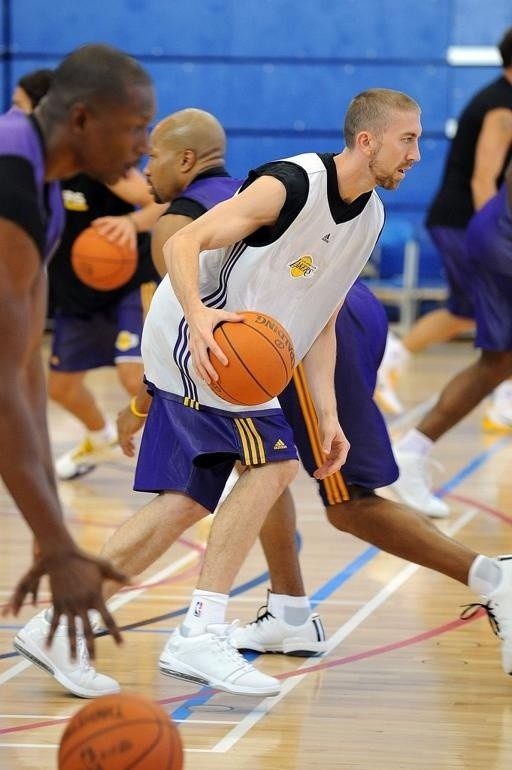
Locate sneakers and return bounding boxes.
[483,555,512,675]
[159,613,330,697]
[12,608,122,699]
[53,436,120,480]
[371,365,512,518]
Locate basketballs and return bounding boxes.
[57,692,185,770]
[70,227,138,289]
[206,311,295,406]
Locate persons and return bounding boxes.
[368,32,512,434]
[4,70,512,699]
[2,41,159,655]
